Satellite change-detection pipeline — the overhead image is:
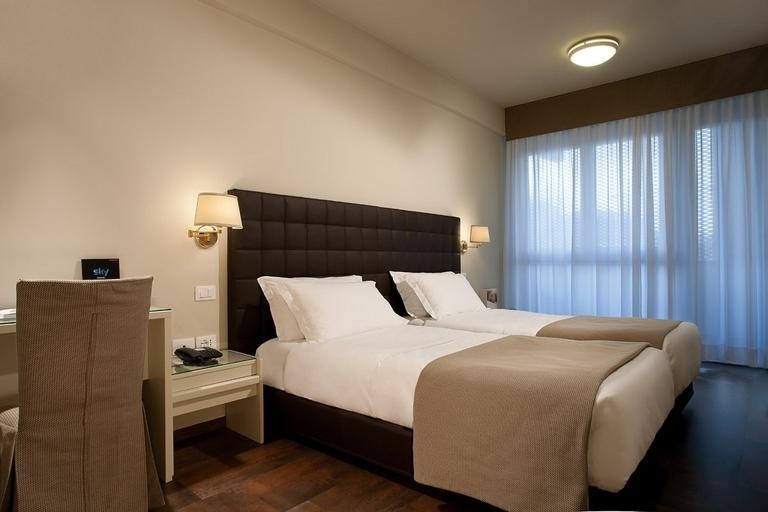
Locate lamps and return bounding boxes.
[568,35,621,68]
[183,189,246,249]
[460,224,491,252]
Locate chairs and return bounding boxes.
[14,277,155,512]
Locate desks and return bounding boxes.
[170,348,264,477]
[0,302,177,495]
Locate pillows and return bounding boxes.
[405,272,487,320]
[257,274,362,341]
[389,270,455,318]
[276,280,409,344]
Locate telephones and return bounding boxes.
[175,347,223,363]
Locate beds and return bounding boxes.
[405,309,702,439]
[256,324,676,511]
